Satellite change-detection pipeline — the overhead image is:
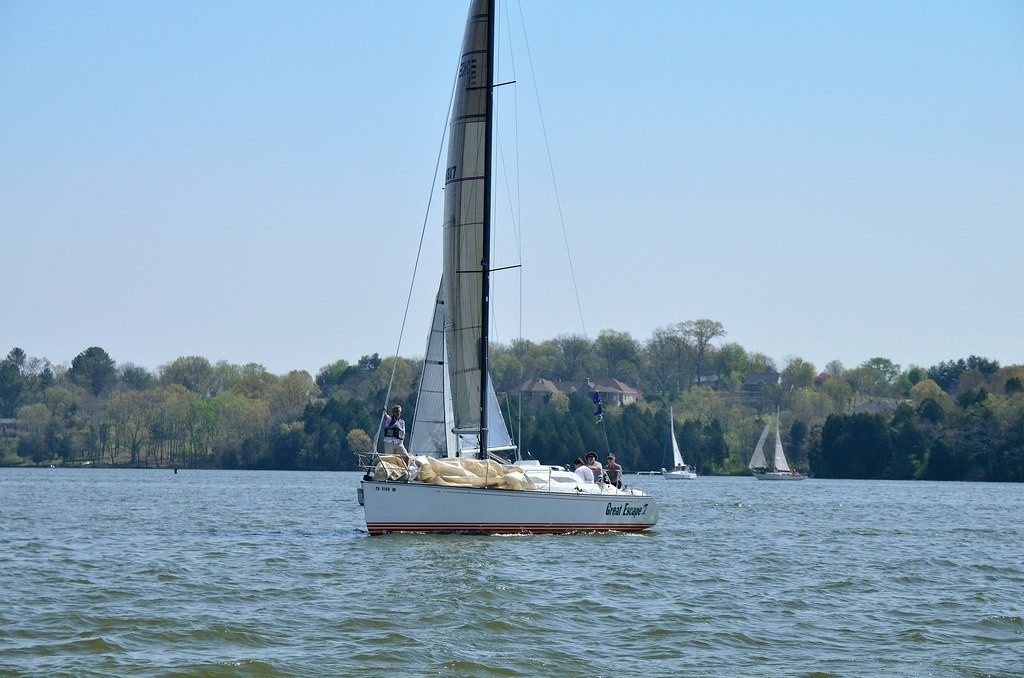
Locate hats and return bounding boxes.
[606,452,615,459]
[585,451,598,461]
[573,458,586,465]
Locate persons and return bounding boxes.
[604,453,622,489]
[585,451,603,483]
[383,405,408,455]
[792,469,797,476]
[573,458,594,484]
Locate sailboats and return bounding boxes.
[660,406,697,480]
[358,0,658,536]
[748,405,807,480]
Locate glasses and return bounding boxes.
[394,410,402,414]
[588,455,594,458]
